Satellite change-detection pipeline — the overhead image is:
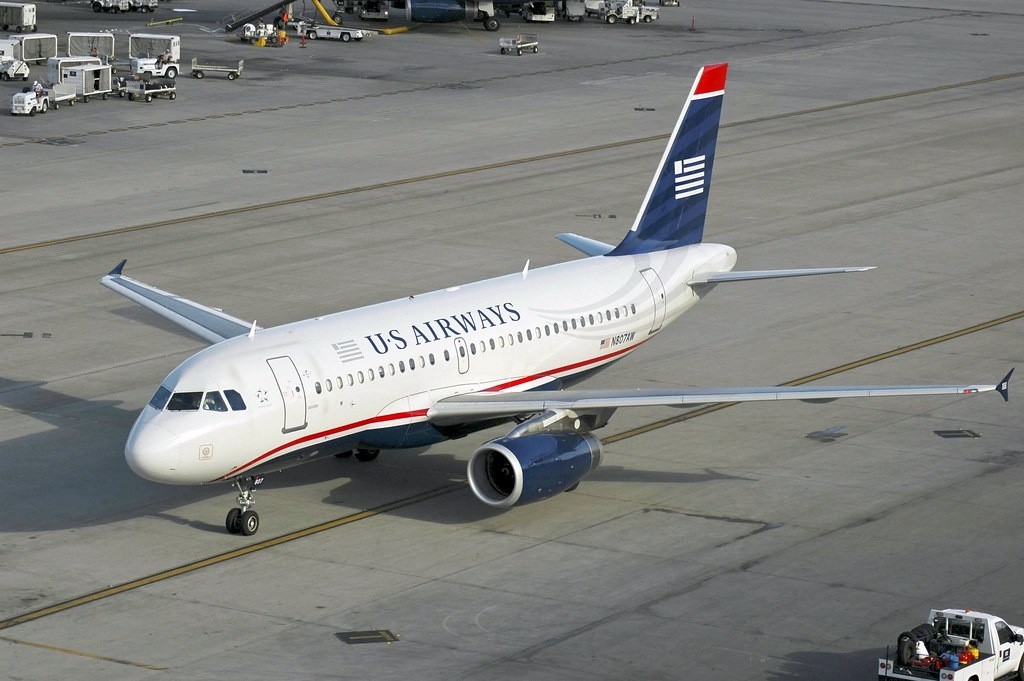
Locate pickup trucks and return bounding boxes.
[876,606,1024,681]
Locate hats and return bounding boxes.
[166,49,169,52]
[33,81,37,85]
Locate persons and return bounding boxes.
[32,81,44,100]
[89,48,98,56]
[156,49,172,68]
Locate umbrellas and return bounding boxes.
[282,12,289,22]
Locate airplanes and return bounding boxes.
[96,59,1018,538]
